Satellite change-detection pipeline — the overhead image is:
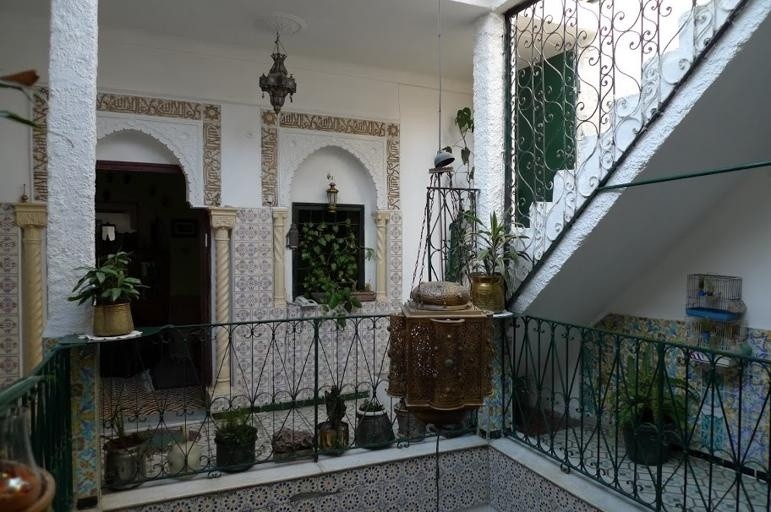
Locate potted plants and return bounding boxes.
[66,251,152,336]
[316,384,350,455]
[468,211,530,313]
[214,405,258,473]
[296,221,378,315]
[102,394,150,492]
[608,347,700,464]
[356,397,395,450]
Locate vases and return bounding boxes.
[393,405,426,442]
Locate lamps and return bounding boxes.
[326,173,340,212]
[258,34,296,116]
